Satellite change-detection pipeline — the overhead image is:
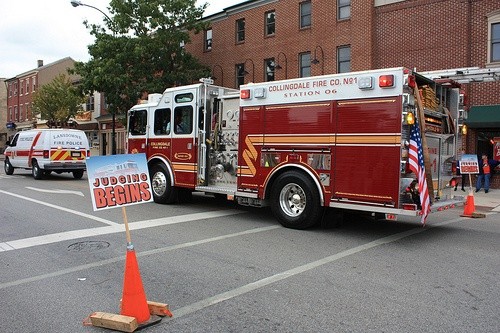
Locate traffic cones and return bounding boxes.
[461,191,477,218]
[119,245,163,332]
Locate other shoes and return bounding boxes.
[475,189,480,192]
[461,188,465,192]
[484,189,488,193]
[454,188,457,191]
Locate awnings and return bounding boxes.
[464,106,500,127]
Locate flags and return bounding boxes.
[408,112,431,224]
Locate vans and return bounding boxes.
[4,128,91,180]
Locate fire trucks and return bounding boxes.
[123,65,465,234]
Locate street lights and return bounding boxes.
[70,0,118,156]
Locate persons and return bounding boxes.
[475,152,500,194]
[453,150,467,192]
